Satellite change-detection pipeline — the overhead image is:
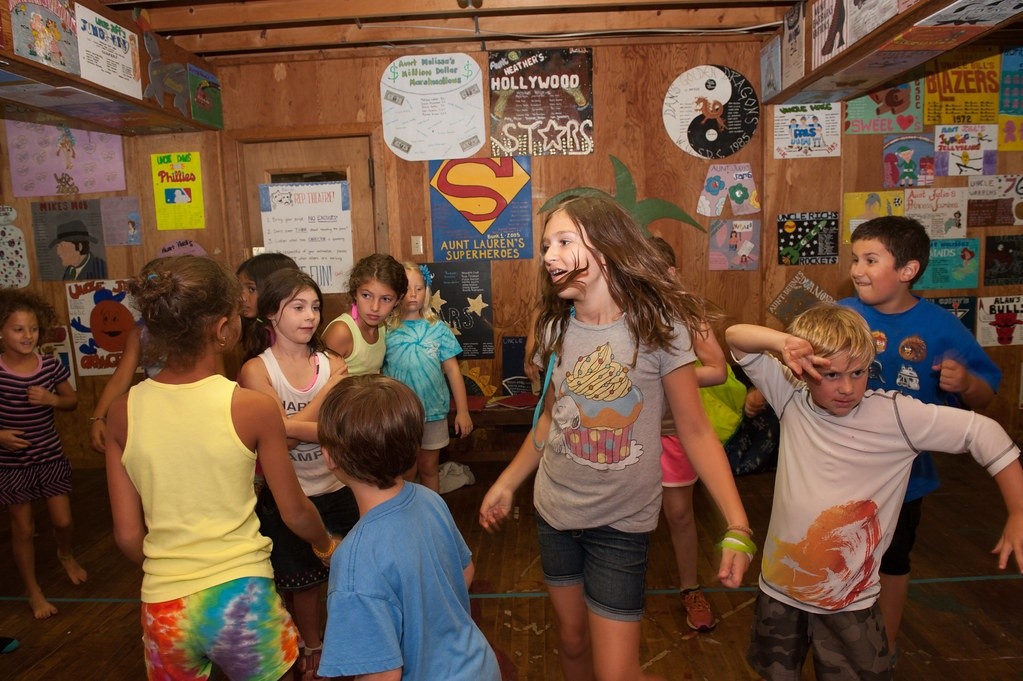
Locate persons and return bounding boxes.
[746,216,1002,670]
[0,252,503,681]
[480,195,756,681]
[725,301,1023,681]
[629,236,725,635]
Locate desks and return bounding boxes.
[447,395,537,428]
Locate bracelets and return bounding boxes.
[720,525,754,560]
[88,415,106,423]
[312,535,337,559]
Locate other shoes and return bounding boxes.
[296,641,330,681]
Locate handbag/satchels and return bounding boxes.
[692,356,746,446]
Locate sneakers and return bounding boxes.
[680,584,716,632]
